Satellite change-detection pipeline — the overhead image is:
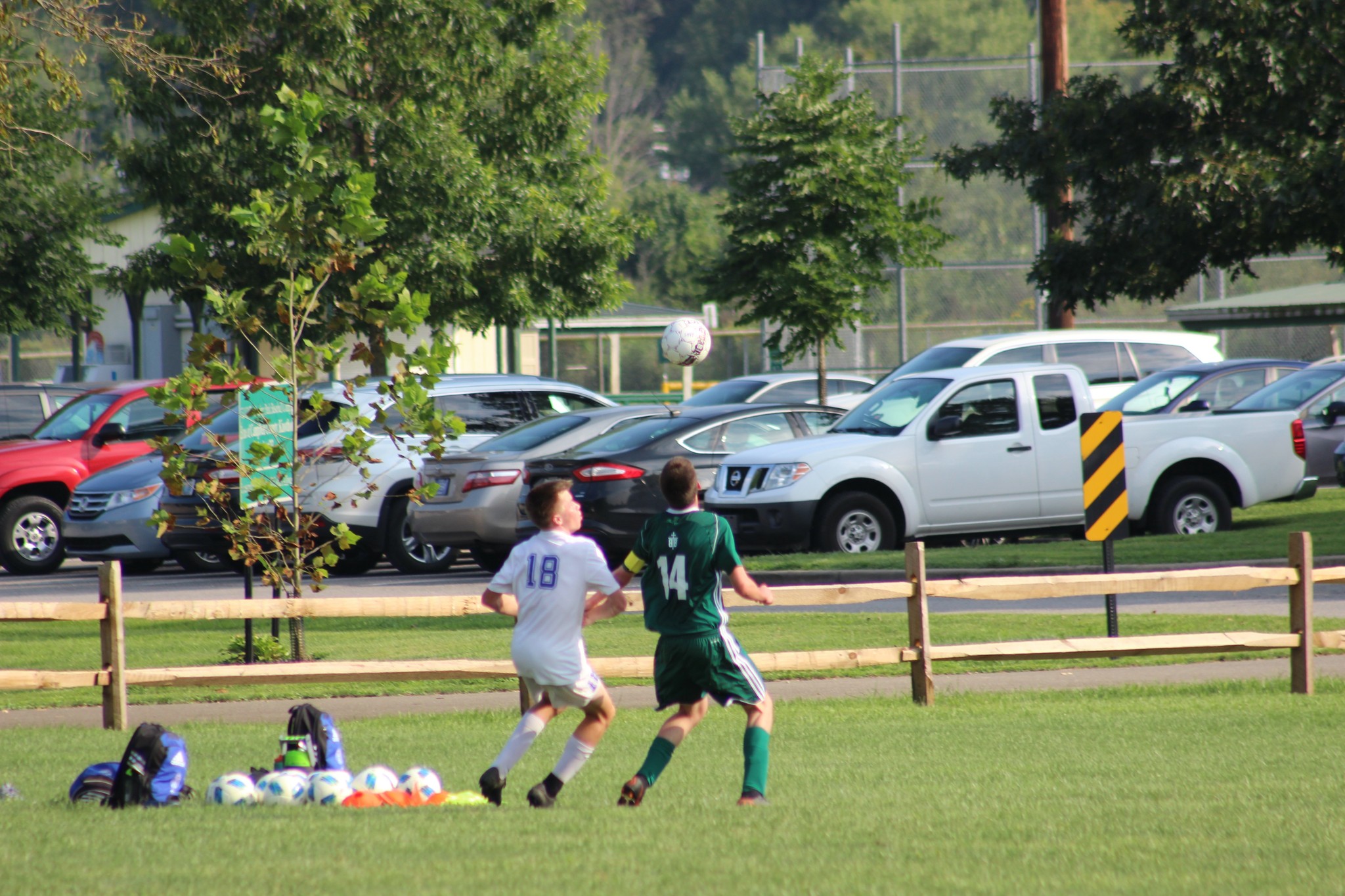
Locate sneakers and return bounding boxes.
[526,783,555,809]
[617,775,647,806]
[479,766,505,807]
[737,790,768,806]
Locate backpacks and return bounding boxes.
[108,722,187,810]
[69,762,197,807]
[288,704,346,770]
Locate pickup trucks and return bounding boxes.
[705,361,1320,560]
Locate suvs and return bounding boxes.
[59,375,622,577]
[0,372,279,578]
[825,328,1244,429]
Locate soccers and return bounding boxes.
[660,316,712,368]
[210,764,445,811]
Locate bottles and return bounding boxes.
[274,755,284,771]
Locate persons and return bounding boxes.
[478,480,627,809]
[584,457,774,807]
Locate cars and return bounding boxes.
[512,402,894,579]
[1095,359,1308,416]
[407,403,785,574]
[681,373,929,413]
[1228,355,1345,489]
